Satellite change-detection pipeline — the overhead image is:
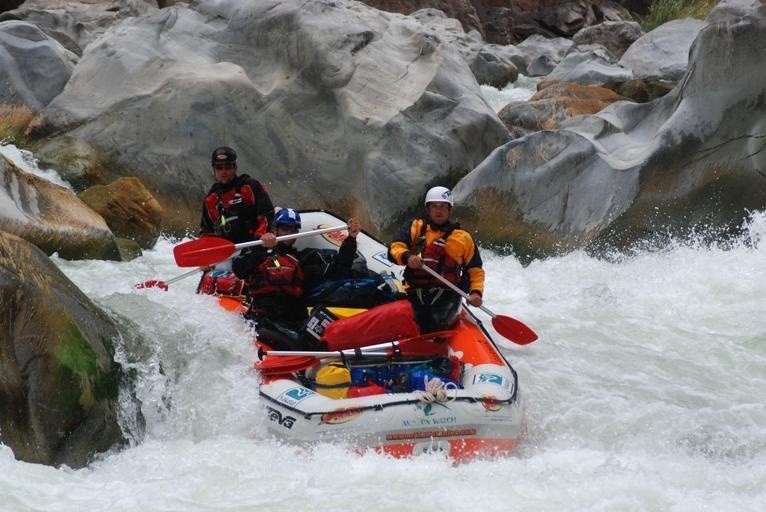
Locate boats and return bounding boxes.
[191,211,528,467]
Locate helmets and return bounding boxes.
[271,207,301,228]
[425,187,453,207]
[212,147,237,166]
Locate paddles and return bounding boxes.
[422,264,538,345]
[174,225,351,267]
[255,331,449,376]
[134,247,252,291]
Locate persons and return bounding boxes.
[230,207,363,350]
[199,145,275,296]
[386,185,484,341]
